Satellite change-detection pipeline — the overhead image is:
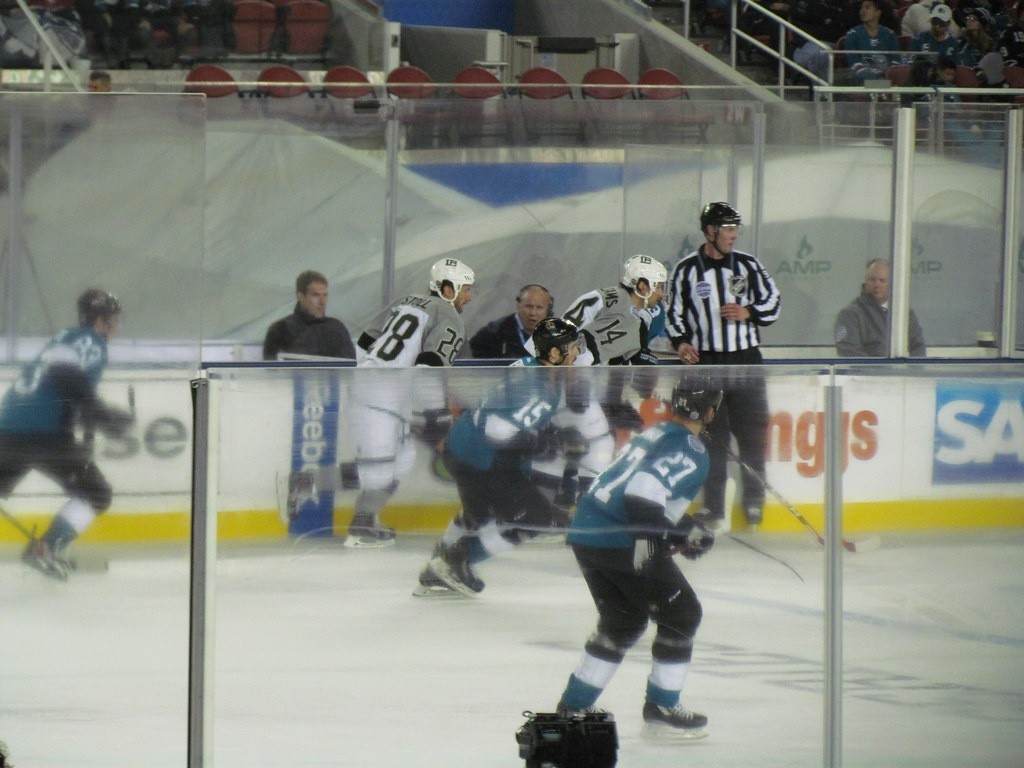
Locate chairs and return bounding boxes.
[152,0,714,145]
[754,0,1024,103]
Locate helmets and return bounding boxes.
[532,317,579,365]
[671,374,725,423]
[700,202,742,243]
[621,255,669,295]
[429,257,475,302]
[75,287,120,329]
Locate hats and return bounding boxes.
[971,7,991,26]
[929,4,952,22]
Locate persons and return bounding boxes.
[262,270,356,359]
[753,0,1024,146]
[343,258,475,549]
[834,259,926,358]
[555,374,724,740]
[664,202,782,533]
[75,0,237,67]
[413,317,580,601]
[0,289,132,580]
[553,254,668,510]
[468,285,554,358]
[88,72,110,92]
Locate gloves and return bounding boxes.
[566,381,592,414]
[556,425,591,456]
[424,410,453,442]
[630,351,662,400]
[520,434,559,461]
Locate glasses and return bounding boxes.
[966,17,980,23]
[930,19,949,28]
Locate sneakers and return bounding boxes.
[743,496,764,532]
[412,565,464,597]
[556,704,613,722]
[640,699,709,743]
[20,525,77,581]
[341,513,395,547]
[693,508,730,538]
[429,550,485,597]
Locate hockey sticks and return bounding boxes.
[126,383,138,422]
[722,476,737,531]
[1,506,110,582]
[554,452,807,585]
[700,431,882,554]
